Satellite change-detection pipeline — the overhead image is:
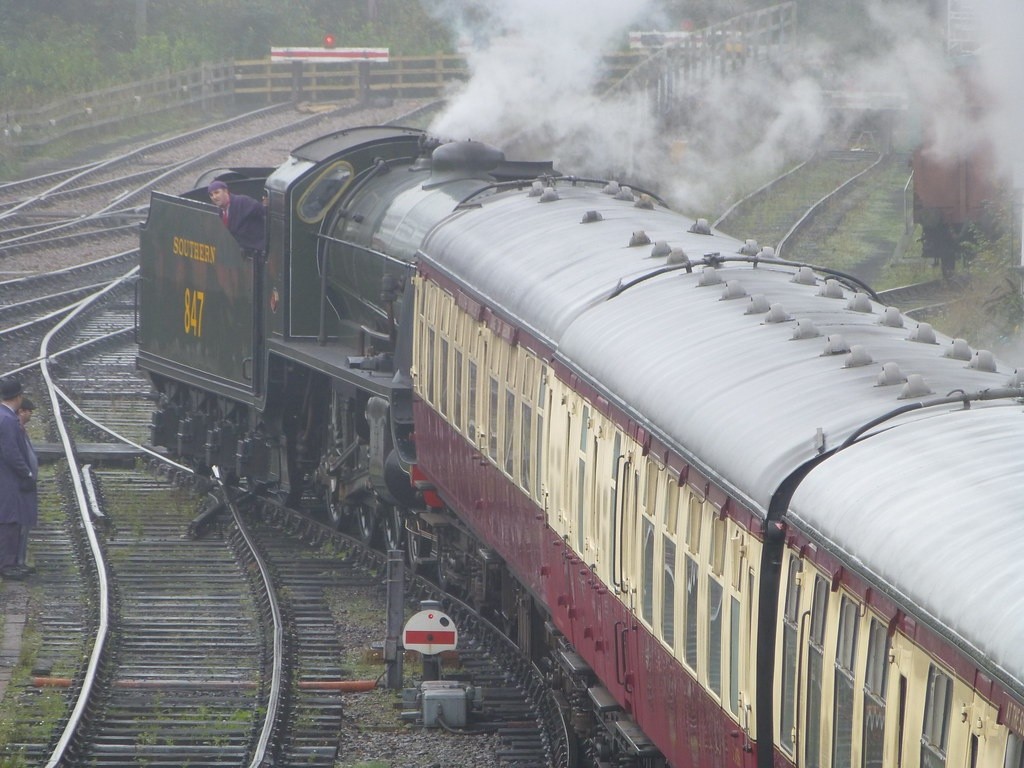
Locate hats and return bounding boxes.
[20,398,37,410]
[207,180,228,194]
[0,379,22,399]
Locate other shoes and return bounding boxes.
[0,569,25,581]
[17,564,36,574]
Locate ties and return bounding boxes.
[224,211,228,224]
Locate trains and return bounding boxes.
[131,122,1024,768]
[910,82,1022,281]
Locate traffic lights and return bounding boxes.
[322,33,337,50]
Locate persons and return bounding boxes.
[0,375,40,580]
[208,180,266,259]
